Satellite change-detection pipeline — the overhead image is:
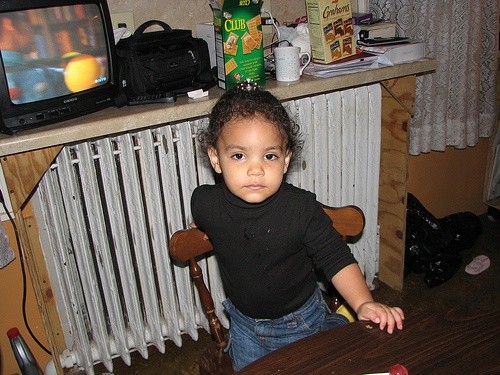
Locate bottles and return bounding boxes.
[7,327,44,375]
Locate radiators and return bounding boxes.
[29,82,381,375]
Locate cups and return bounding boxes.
[274,47,310,81]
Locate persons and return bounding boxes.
[0,10,71,107]
[189,82,405,372]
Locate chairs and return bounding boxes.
[168,205,365,375]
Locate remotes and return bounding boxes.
[129,92,177,106]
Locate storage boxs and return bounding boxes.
[304,0,355,62]
[209,0,266,91]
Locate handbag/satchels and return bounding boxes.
[116,20,218,108]
[404,190,457,287]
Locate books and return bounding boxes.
[305,20,426,78]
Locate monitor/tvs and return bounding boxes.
[0,0,119,136]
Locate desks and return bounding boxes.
[0,57,439,375]
[232,309,500,375]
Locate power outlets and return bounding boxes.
[111,12,135,34]
